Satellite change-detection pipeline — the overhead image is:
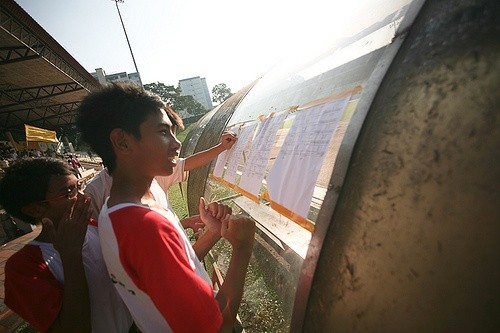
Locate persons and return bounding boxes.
[77,84,256,333]
[0,141,83,183]
[0,157,134,332]
[82,106,239,220]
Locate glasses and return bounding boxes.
[33,178,87,205]
